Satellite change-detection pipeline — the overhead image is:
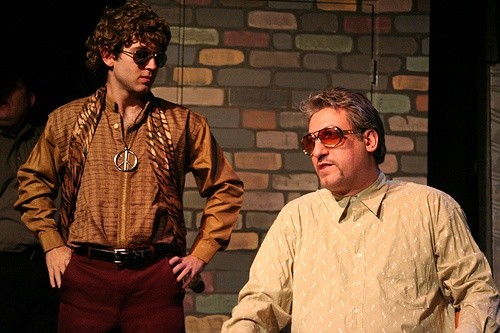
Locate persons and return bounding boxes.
[221,88,500,333]
[12,0,244,333]
[0,69,61,333]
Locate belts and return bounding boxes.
[74,246,167,266]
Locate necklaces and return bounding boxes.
[113,106,144,172]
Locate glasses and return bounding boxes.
[301,126,363,155]
[119,47,169,68]
